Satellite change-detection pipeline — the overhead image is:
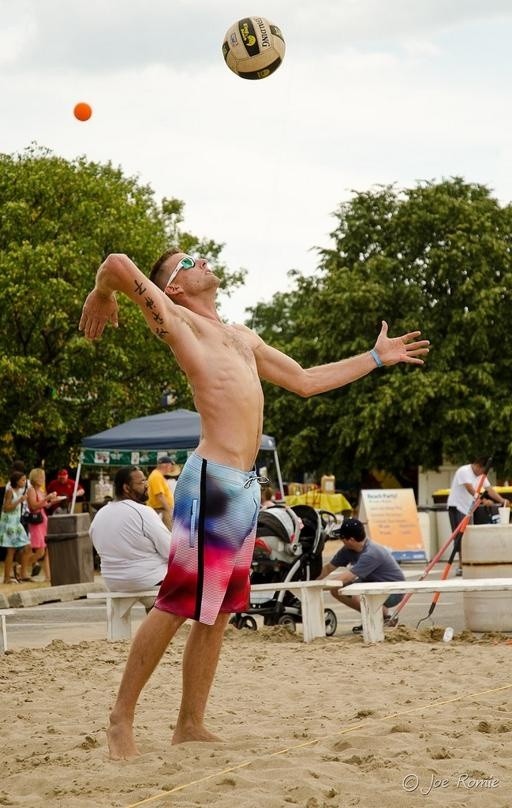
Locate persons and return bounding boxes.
[84,465,172,614]
[445,457,512,576]
[317,519,407,634]
[141,458,175,532]
[77,246,433,752]
[0,466,86,583]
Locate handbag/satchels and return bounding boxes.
[20,516,28,527]
[28,512,43,523]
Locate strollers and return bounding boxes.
[229,502,351,640]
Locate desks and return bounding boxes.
[282,491,353,527]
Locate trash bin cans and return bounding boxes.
[45,513,93,586]
[457,520,512,634]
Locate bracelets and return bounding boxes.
[372,348,383,370]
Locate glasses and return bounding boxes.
[163,254,201,297]
[134,478,148,484]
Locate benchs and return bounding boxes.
[336,577,512,646]
[84,579,344,644]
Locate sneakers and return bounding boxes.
[352,625,363,634]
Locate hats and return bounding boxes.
[165,460,181,476]
[59,469,68,478]
[331,519,363,536]
[159,457,175,465]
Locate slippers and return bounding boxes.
[22,575,37,582]
[14,564,22,583]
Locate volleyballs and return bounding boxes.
[75,102,92,121]
[223,16,286,79]
